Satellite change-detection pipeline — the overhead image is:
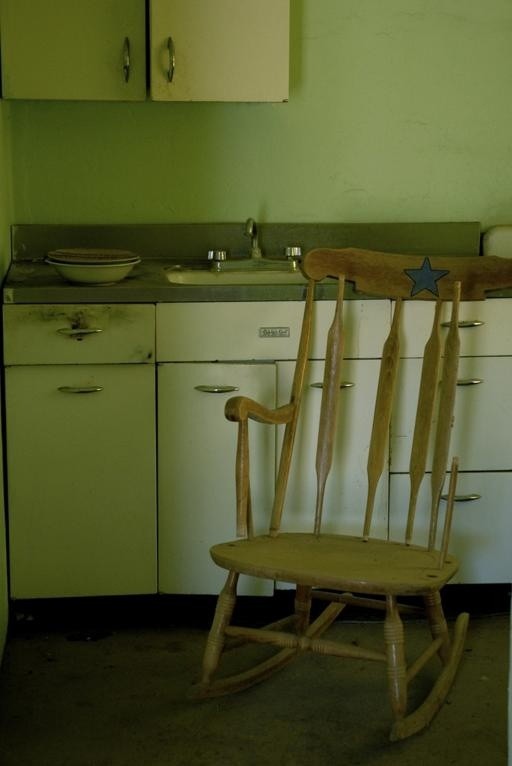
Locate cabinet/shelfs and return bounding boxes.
[159,302,389,602]
[1,3,290,104]
[4,306,156,604]
[391,299,511,595]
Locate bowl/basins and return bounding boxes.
[45,249,142,286]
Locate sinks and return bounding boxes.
[165,268,343,286]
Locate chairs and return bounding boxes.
[186,244,510,725]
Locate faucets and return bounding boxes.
[244,216,265,261]
